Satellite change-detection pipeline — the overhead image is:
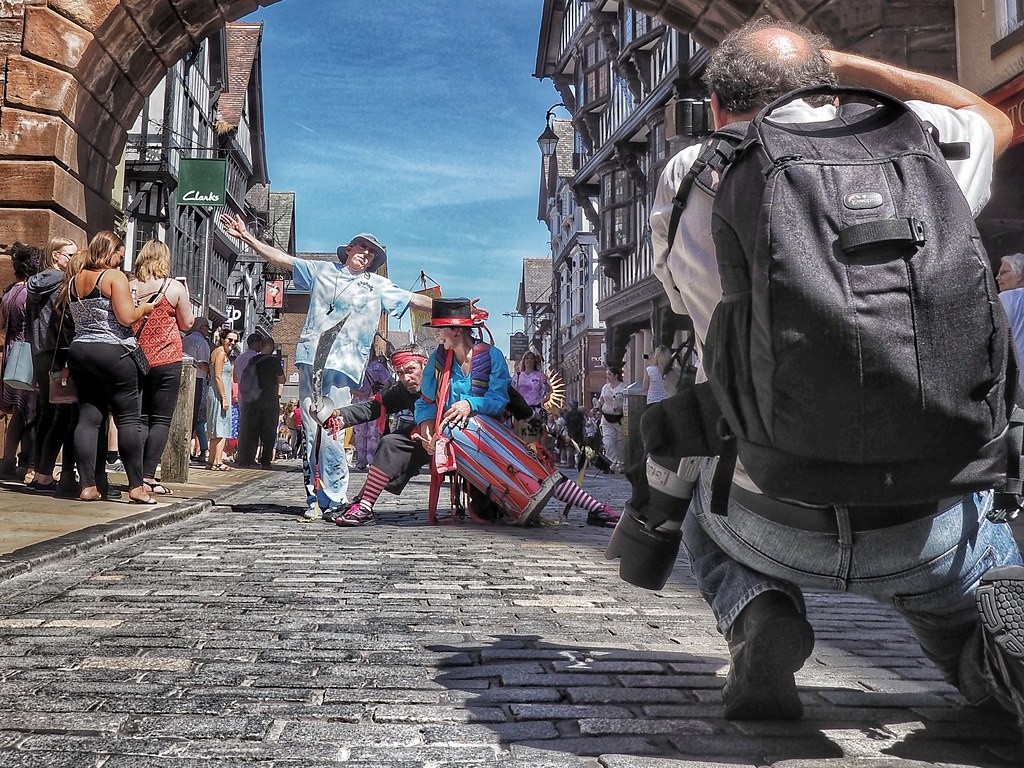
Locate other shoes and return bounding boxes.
[26,478,58,493]
[976,565,1024,729]
[0,454,27,479]
[721,593,815,720]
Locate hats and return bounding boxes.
[421,296,495,346]
[568,400,579,409]
[337,233,387,273]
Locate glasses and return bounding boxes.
[997,269,1014,276]
[227,337,239,344]
[356,240,376,257]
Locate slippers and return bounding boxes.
[80,486,102,502]
[130,487,156,504]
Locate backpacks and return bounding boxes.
[666,82,1024,523]
[238,355,274,402]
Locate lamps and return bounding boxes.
[537,104,581,157]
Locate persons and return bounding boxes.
[218,213,488,519]
[0,231,195,505]
[995,252,1024,388]
[351,346,393,470]
[181,316,287,470]
[546,400,603,469]
[510,351,553,442]
[323,343,541,527]
[633,14,1024,736]
[590,366,626,474]
[271,398,304,459]
[334,298,621,528]
[642,345,676,406]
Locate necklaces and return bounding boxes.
[329,264,356,311]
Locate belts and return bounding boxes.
[727,480,966,535]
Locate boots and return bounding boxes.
[105,471,122,498]
[54,470,81,499]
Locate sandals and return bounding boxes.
[144,480,174,495]
[204,462,235,471]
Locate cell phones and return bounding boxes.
[642,354,649,359]
[147,294,160,304]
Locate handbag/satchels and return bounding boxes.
[601,410,623,425]
[130,345,150,375]
[49,367,78,404]
[2,341,37,391]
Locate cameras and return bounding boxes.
[605,450,706,591]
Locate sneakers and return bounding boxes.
[304,498,340,519]
[586,504,621,528]
[332,497,376,526]
[105,459,126,474]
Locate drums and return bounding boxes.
[438,413,564,527]
